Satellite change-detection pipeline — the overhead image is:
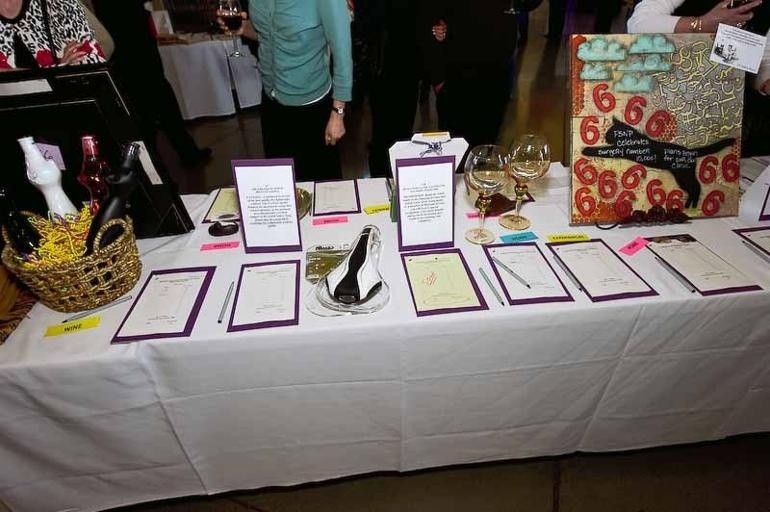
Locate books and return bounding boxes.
[312,179,361,217]
[546,237,659,303]
[227,259,301,332]
[110,264,217,342]
[642,233,764,296]
[202,186,241,223]
[230,158,303,254]
[394,155,456,251]
[730,185,770,256]
[400,247,489,317]
[483,241,574,306]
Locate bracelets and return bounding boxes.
[687,15,704,32]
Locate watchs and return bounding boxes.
[331,106,347,114]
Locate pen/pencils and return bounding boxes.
[552,255,583,293]
[742,238,769,263]
[478,266,506,307]
[491,256,532,292]
[654,256,695,295]
[60,295,134,325]
[216,280,234,323]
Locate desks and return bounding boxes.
[1,154,764,509]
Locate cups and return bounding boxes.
[499,134,550,230]
[219,1,245,58]
[463,144,507,243]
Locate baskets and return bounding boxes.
[1,210,143,313]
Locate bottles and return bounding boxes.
[80,140,141,287]
[17,135,81,223]
[0,188,42,261]
[75,135,112,216]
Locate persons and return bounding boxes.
[350,0,449,178]
[214,0,354,177]
[754,27,770,95]
[625,0,763,33]
[92,0,212,167]
[0,0,115,73]
[411,0,544,174]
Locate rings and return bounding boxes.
[736,21,743,29]
